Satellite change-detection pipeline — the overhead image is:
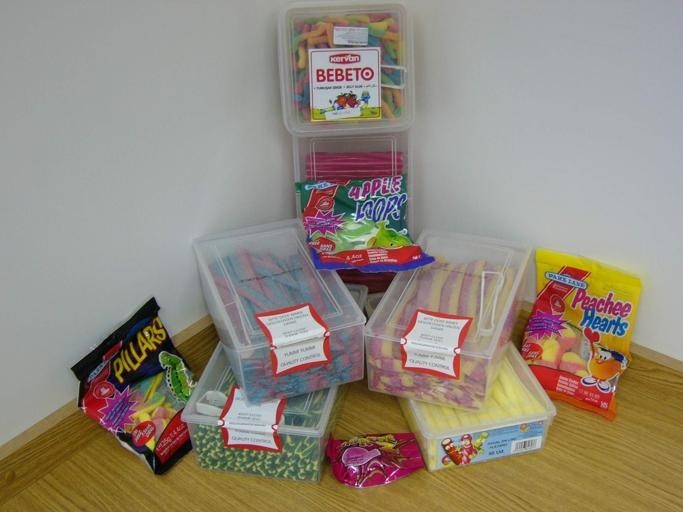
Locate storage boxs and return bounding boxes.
[277,6,419,261]
[363,227,535,412]
[191,219,368,409]
[178,284,368,481]
[365,291,557,475]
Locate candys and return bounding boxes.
[129,12,587,481]
